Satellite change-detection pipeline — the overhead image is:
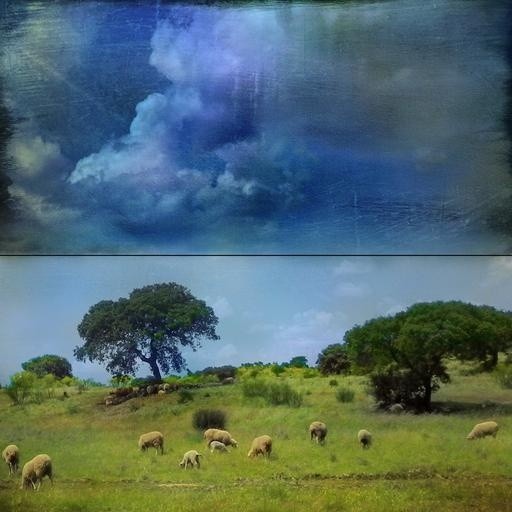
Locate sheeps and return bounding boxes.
[19,454,54,492]
[103,380,172,407]
[309,421,327,444]
[179,449,201,471]
[247,435,273,462]
[138,430,165,456]
[465,420,499,441]
[204,428,239,455]
[1,444,20,477]
[357,429,373,450]
